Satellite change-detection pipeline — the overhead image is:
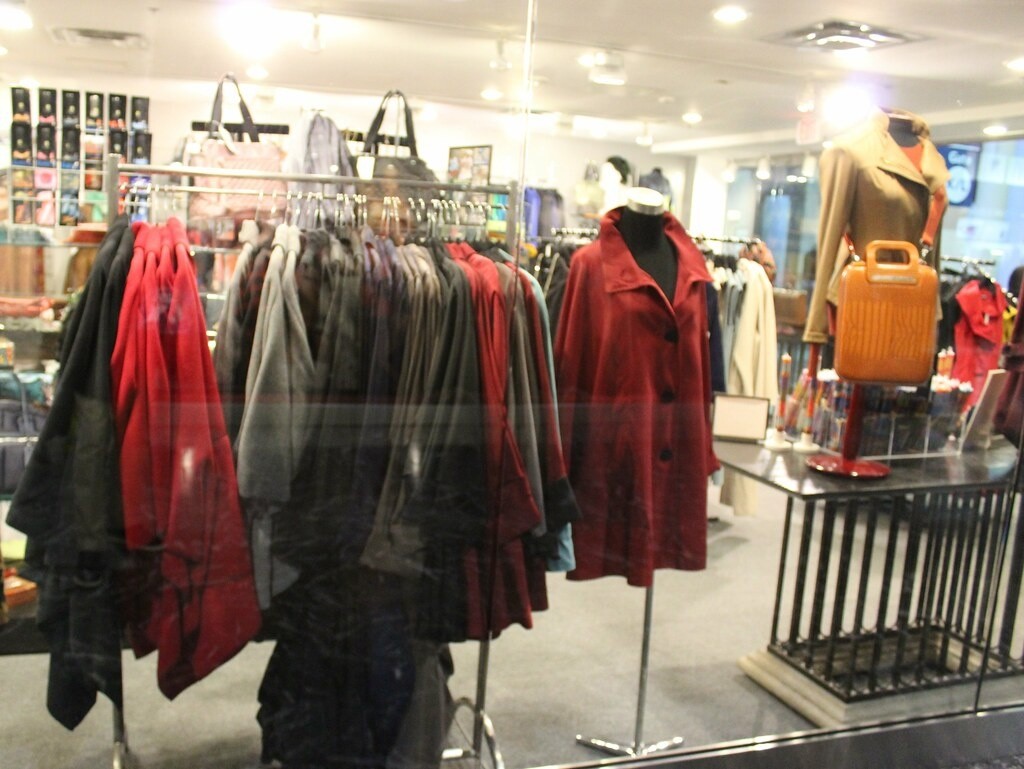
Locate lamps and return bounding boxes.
[754,157,774,180]
[599,148,665,208]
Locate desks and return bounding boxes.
[714,428,1023,732]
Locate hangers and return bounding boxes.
[934,254,998,297]
[539,227,764,276]
[111,180,517,255]
[336,132,408,165]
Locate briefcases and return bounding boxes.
[834,240,939,386]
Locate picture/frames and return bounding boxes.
[445,145,492,225]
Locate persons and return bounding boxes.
[601,157,631,218]
[641,166,677,212]
[803,104,948,343]
[554,186,721,588]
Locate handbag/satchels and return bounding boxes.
[188,73,288,222]
[348,90,441,238]
